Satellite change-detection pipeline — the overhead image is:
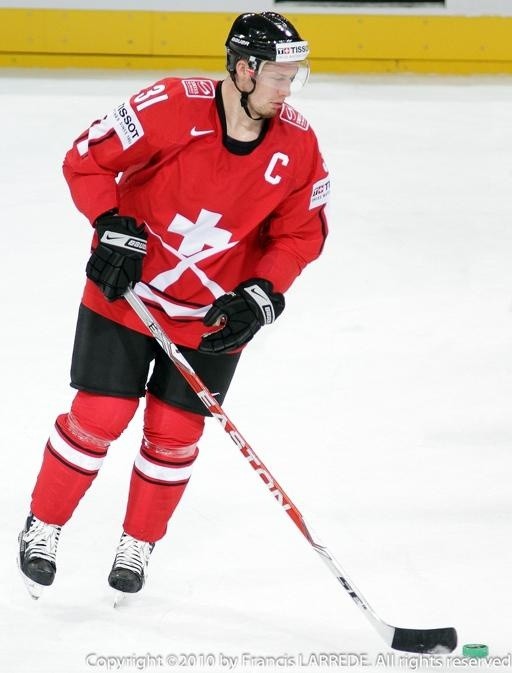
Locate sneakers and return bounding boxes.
[108,530,155,593]
[18,511,61,585]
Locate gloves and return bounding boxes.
[198,278,285,356]
[86,216,148,302]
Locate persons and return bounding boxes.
[18,10,330,610]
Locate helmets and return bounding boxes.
[225,11,309,95]
